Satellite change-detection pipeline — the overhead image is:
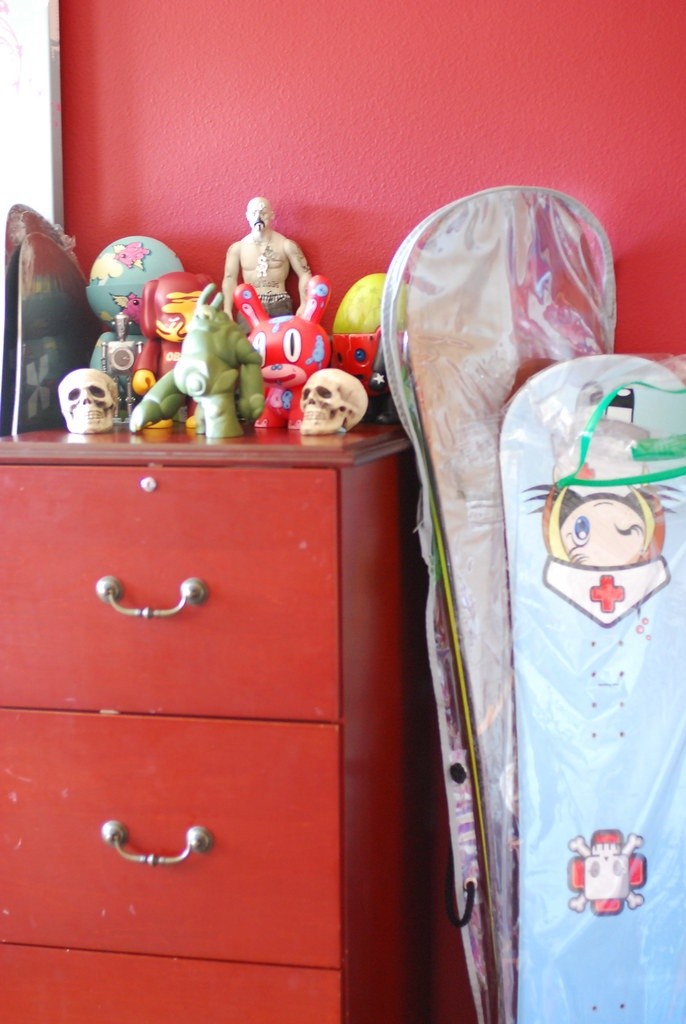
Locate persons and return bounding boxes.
[100,312,144,419]
[221,197,312,336]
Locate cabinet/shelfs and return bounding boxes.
[1,427,413,1024]
